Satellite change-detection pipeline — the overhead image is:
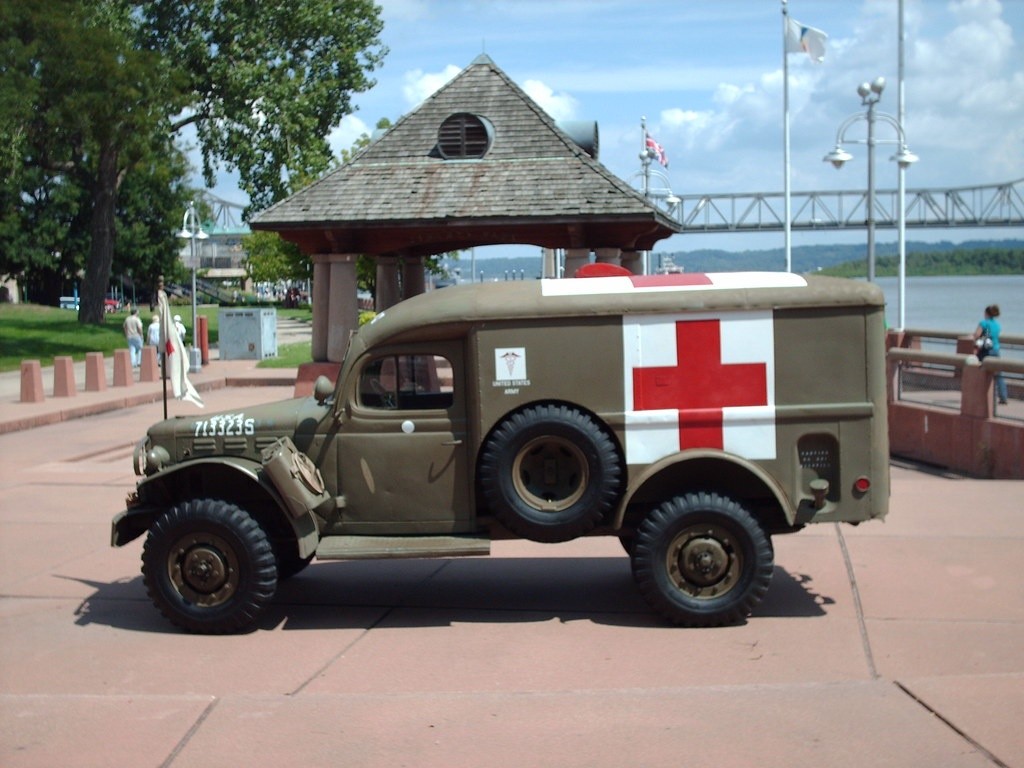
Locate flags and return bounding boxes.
[158,290,204,409]
[645,133,669,171]
[786,16,828,64]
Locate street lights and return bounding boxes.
[176,203,201,371]
[823,76,917,285]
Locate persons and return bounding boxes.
[123,308,144,368]
[973,305,1008,404]
[125,296,130,312]
[232,283,287,303]
[173,314,186,343]
[149,297,155,312]
[146,314,161,367]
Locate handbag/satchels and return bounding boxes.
[975,329,993,349]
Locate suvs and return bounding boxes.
[109,270,889,636]
